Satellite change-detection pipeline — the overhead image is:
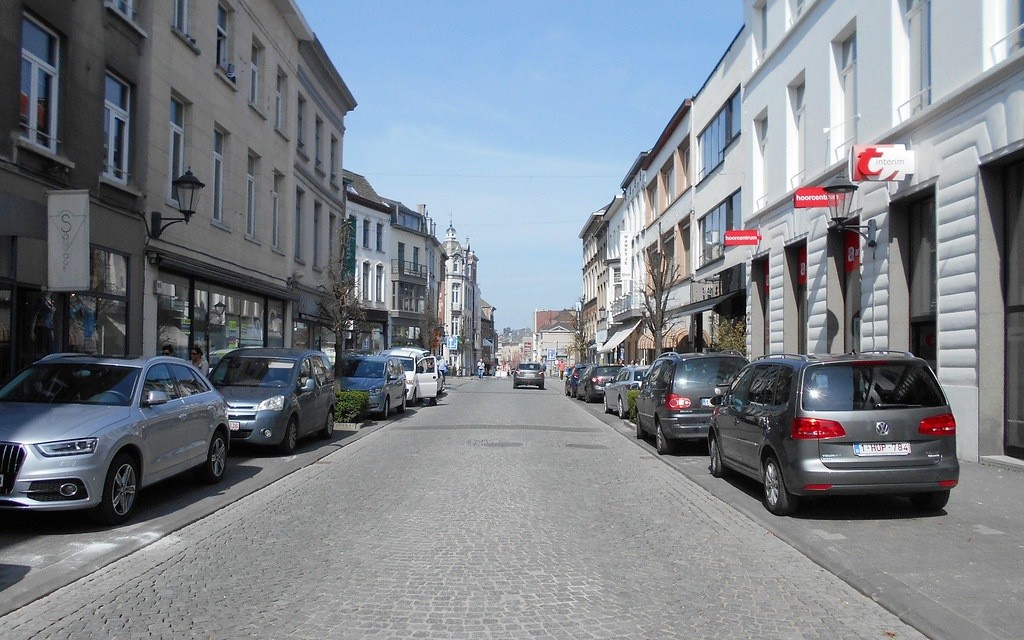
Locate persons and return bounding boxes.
[425,362,441,407]
[187,348,209,377]
[161,345,175,357]
[31,289,99,358]
[537,361,547,377]
[477,358,485,380]
[558,361,565,381]
[489,369,493,376]
[445,360,463,377]
[506,370,511,376]
[617,357,644,366]
[437,356,447,384]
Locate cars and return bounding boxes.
[206,347,339,454]
[511,362,546,389]
[563,363,593,399]
[602,364,652,419]
[377,346,445,408]
[575,362,628,403]
[0,352,232,526]
[329,355,408,421]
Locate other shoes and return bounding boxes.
[432,403,438,406]
[426,403,432,406]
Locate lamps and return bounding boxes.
[211,301,227,323]
[138,166,206,240]
[822,170,878,248]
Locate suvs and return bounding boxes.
[628,346,752,455]
[708,350,960,517]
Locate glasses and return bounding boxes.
[191,353,196,355]
[163,352,169,355]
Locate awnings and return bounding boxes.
[695,328,711,348]
[637,327,688,349]
[663,289,742,321]
[597,318,642,353]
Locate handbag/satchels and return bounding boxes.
[479,366,483,370]
